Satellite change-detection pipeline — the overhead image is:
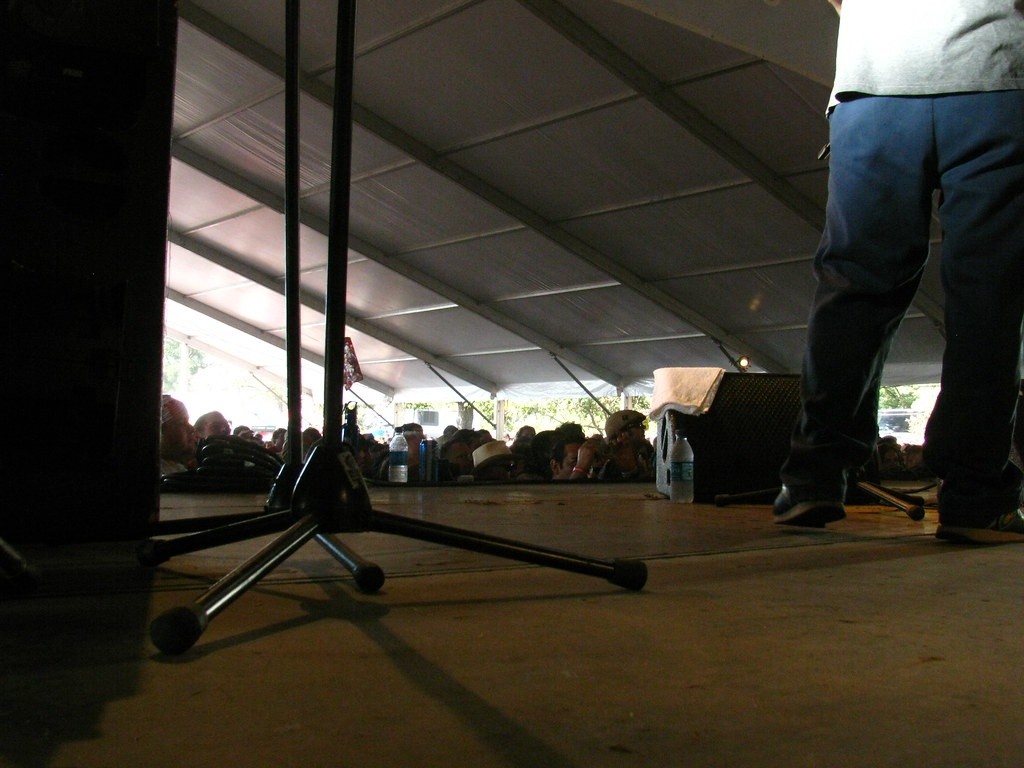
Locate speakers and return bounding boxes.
[657,372,883,503]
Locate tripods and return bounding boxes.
[151,0,650,658]
[712,469,926,520]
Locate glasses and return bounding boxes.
[628,423,647,430]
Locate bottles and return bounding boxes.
[670,429,694,504]
[388,427,409,483]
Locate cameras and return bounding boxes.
[599,444,617,459]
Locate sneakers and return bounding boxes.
[772,470,847,524]
[935,490,1024,543]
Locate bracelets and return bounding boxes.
[571,466,586,474]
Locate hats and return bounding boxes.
[605,410,646,439]
[470,441,525,474]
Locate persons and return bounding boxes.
[774,0,1024,547]
[159,391,1024,485]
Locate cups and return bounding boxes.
[419,439,439,483]
